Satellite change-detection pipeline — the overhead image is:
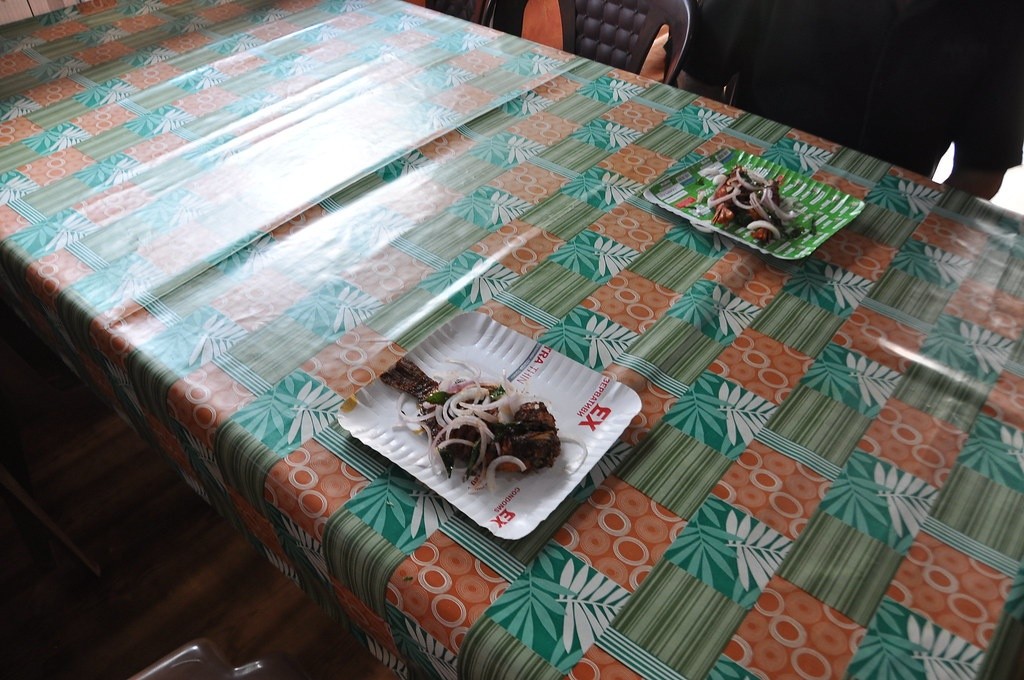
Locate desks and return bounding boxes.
[0,0,1024,680]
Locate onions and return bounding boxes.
[708,167,795,242]
[396,360,588,493]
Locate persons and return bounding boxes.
[671,0,1023,211]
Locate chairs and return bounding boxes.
[481,0,700,85]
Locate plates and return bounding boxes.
[337,313,642,541]
[643,147,866,261]
